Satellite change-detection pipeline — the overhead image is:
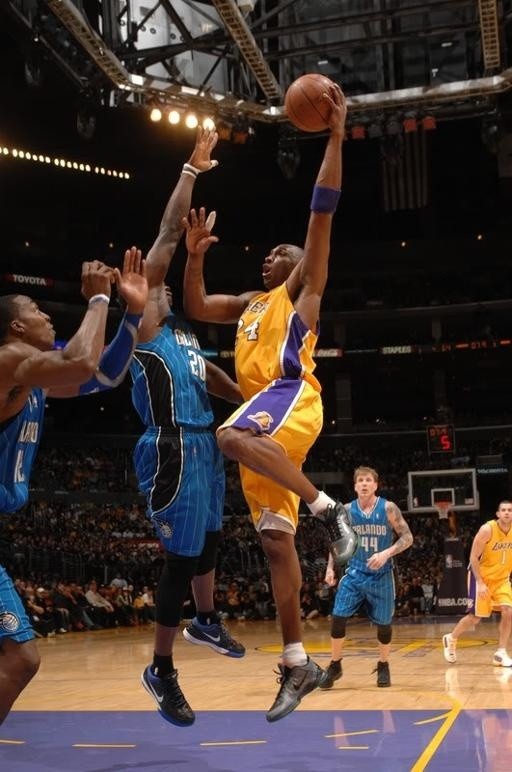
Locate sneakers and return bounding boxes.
[316,502,358,566]
[493,653,512,666]
[140,664,195,726]
[377,661,390,687]
[443,633,456,662]
[318,658,343,689]
[266,656,329,722]
[182,612,245,657]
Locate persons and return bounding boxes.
[2,442,165,637]
[317,465,414,690]
[303,414,505,472]
[214,459,276,620]
[128,125,246,728]
[384,451,481,618]
[294,484,356,620]
[446,668,512,772]
[442,500,511,668]
[0,245,148,726]
[328,711,395,770]
[179,82,348,723]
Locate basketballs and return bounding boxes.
[285,74,336,132]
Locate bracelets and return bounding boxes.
[89,293,111,306]
[182,163,199,179]
